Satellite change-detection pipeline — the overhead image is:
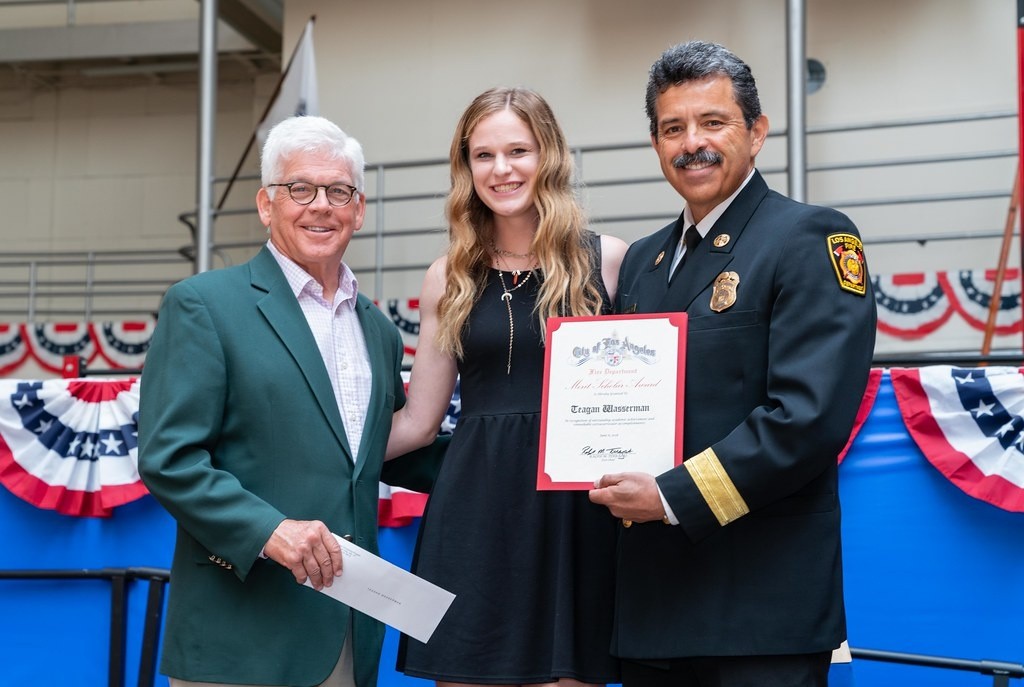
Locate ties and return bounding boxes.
[669,226,704,287]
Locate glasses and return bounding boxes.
[268,180,360,207]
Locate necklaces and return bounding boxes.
[488,240,546,378]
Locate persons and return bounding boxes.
[133,112,453,687]
[585,43,876,687]
[383,87,628,687]
